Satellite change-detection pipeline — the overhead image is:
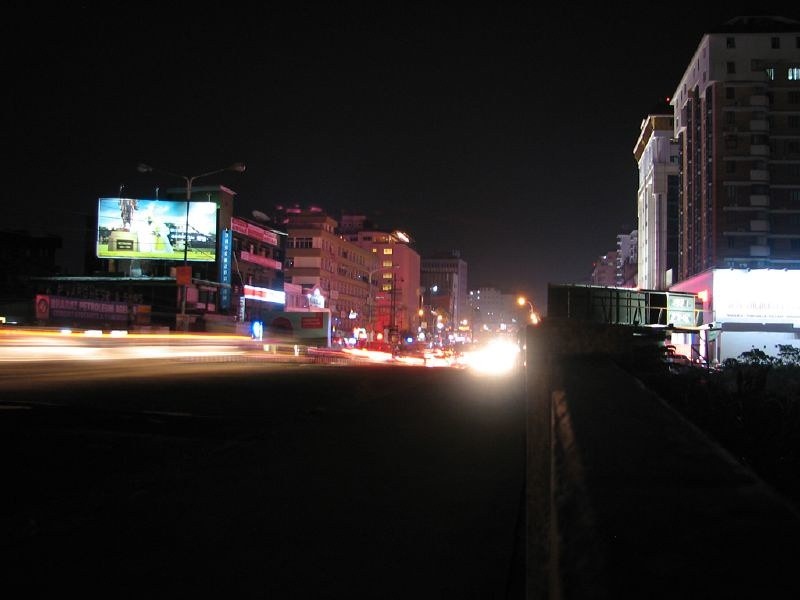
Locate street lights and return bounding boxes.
[516,297,535,315]
[338,263,400,350]
[136,164,247,330]
